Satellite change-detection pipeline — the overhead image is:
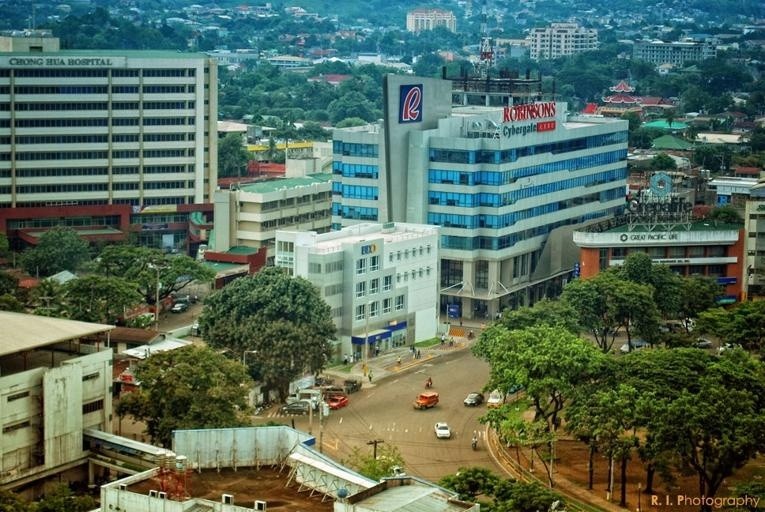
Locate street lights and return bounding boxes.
[363,298,376,377]
[242,348,260,419]
[95,256,111,323]
[147,264,174,334]
[594,433,615,501]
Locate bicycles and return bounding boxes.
[253,399,274,415]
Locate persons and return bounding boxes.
[440,333,445,345]
[409,344,421,360]
[375,344,379,358]
[368,370,373,383]
[342,351,360,365]
[426,376,432,388]
[397,355,402,365]
[471,435,478,447]
[362,362,368,377]
[449,336,454,345]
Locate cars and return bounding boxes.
[163,246,179,254]
[433,421,452,440]
[170,291,195,313]
[279,377,362,415]
[510,381,522,394]
[463,392,484,407]
[587,316,749,356]
[132,313,155,328]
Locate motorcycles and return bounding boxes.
[471,440,477,449]
[423,381,433,388]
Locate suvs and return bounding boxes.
[486,386,509,409]
[412,391,441,410]
